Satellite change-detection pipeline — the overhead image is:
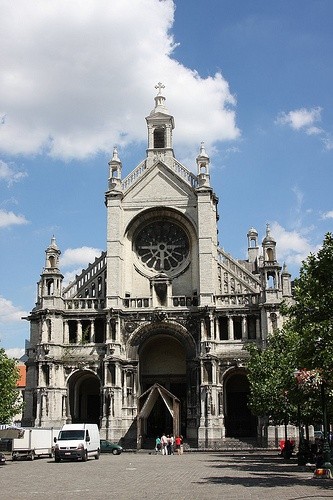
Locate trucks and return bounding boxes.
[12,427,61,461]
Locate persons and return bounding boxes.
[280,432,333,468]
[153,433,184,457]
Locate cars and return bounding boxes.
[0,437,13,452]
[100,439,123,455]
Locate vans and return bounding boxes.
[53,423,101,461]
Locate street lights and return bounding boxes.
[293,369,308,467]
